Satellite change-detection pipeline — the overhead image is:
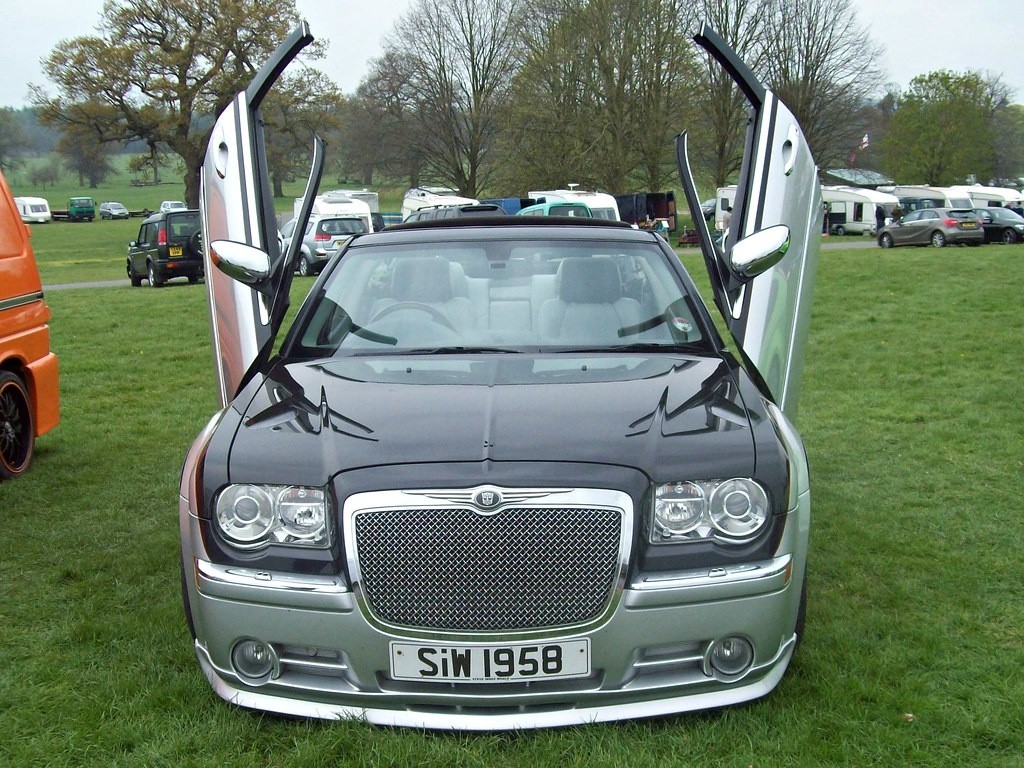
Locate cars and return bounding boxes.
[700,199,716,221]
[967,207,1024,244]
[877,208,985,249]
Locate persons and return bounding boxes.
[875,202,886,239]
[891,203,905,224]
[1013,201,1024,210]
[722,206,732,232]
[1003,201,1013,209]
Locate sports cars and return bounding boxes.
[179,21,826,730]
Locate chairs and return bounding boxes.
[171,225,176,235]
[370,259,476,332]
[338,222,348,233]
[542,258,641,341]
[326,222,337,234]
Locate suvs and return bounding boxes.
[402,203,508,223]
[278,213,369,277]
[126,210,205,288]
[99,201,129,220]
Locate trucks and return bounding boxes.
[50,197,97,222]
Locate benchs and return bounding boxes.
[678,230,700,247]
[130,211,154,217]
[337,178,362,185]
[138,182,154,185]
[448,257,556,335]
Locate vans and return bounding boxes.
[515,202,594,218]
[0,171,60,479]
[160,201,189,213]
